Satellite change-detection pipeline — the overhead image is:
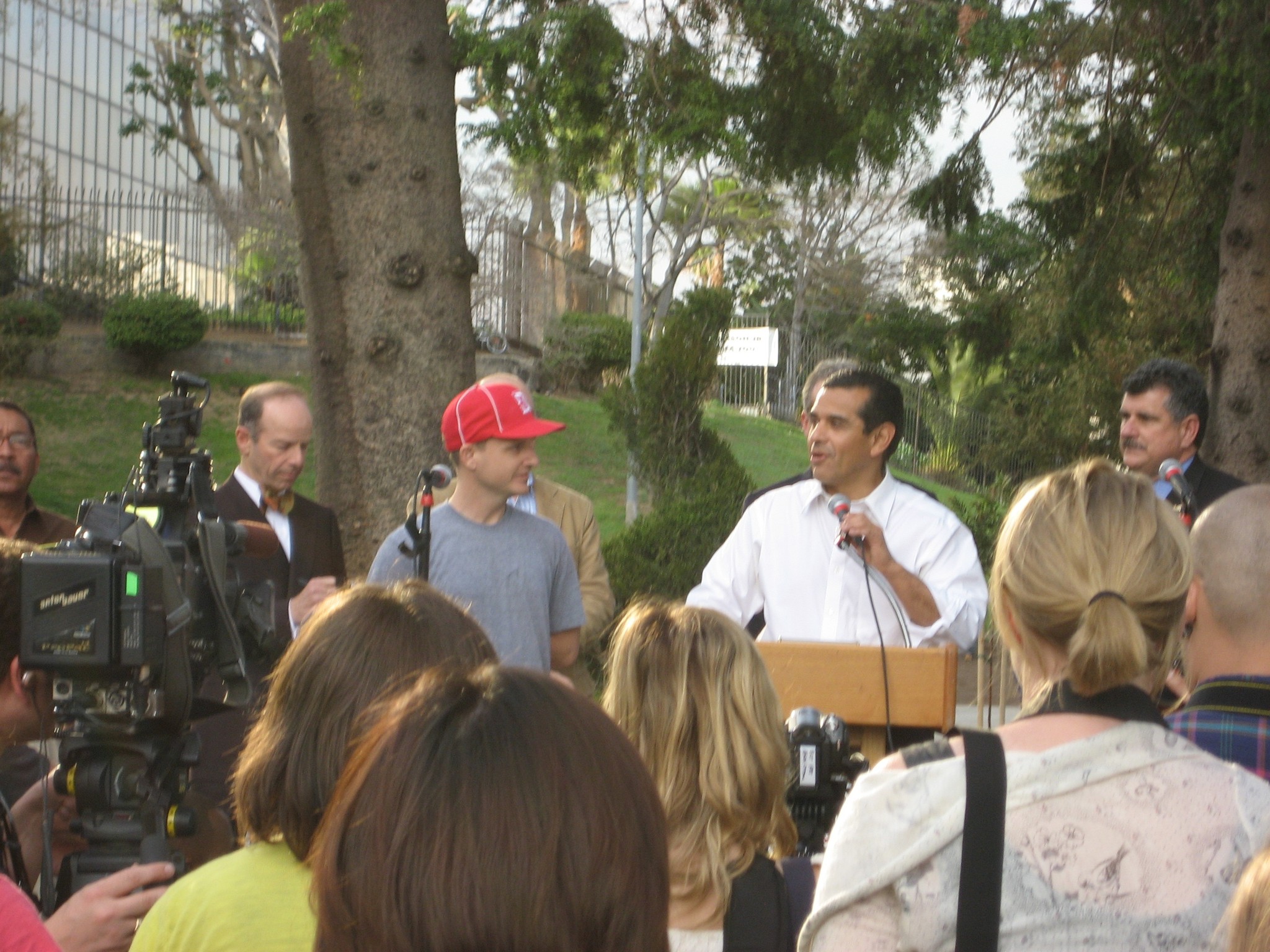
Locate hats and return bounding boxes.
[441,381,567,453]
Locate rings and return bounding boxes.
[134,918,141,935]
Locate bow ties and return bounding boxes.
[260,485,296,515]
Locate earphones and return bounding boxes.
[22,671,37,691]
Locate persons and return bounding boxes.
[128,576,498,952]
[0,382,353,952]
[405,374,615,703]
[601,356,1270,952]
[1118,358,1255,533]
[1161,485,1270,782]
[309,658,671,952]
[365,384,585,675]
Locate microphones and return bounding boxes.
[420,464,452,494]
[828,493,863,549]
[1159,458,1200,519]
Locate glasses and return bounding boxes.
[0,432,34,448]
